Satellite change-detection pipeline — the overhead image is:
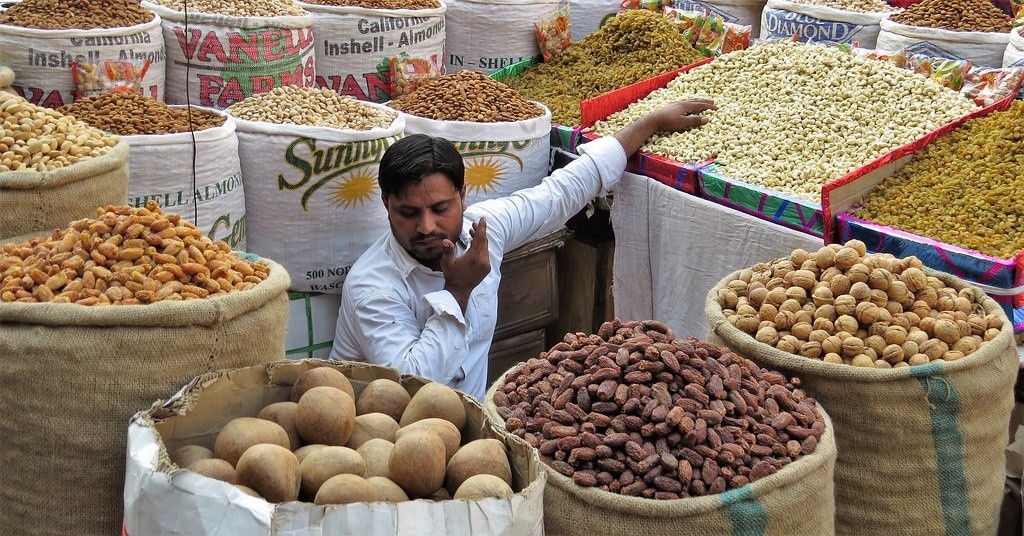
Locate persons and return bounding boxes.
[327,97,720,404]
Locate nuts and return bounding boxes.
[0,0,1024,500]
